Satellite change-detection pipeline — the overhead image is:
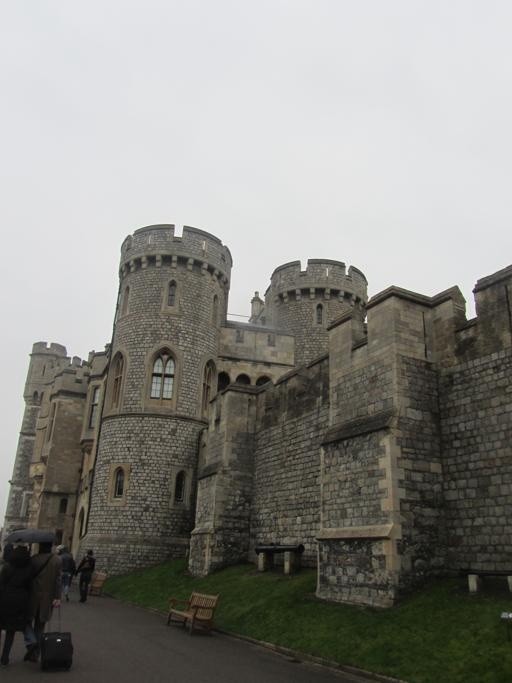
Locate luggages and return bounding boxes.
[41,604,73,671]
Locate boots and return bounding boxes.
[80,592,86,602]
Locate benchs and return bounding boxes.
[168,592,221,635]
[88,572,108,596]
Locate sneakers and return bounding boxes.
[1,656,8,665]
[24,643,40,663]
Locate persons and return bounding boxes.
[1,542,96,667]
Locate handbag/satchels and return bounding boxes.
[79,558,91,572]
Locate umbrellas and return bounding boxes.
[3,527,59,555]
